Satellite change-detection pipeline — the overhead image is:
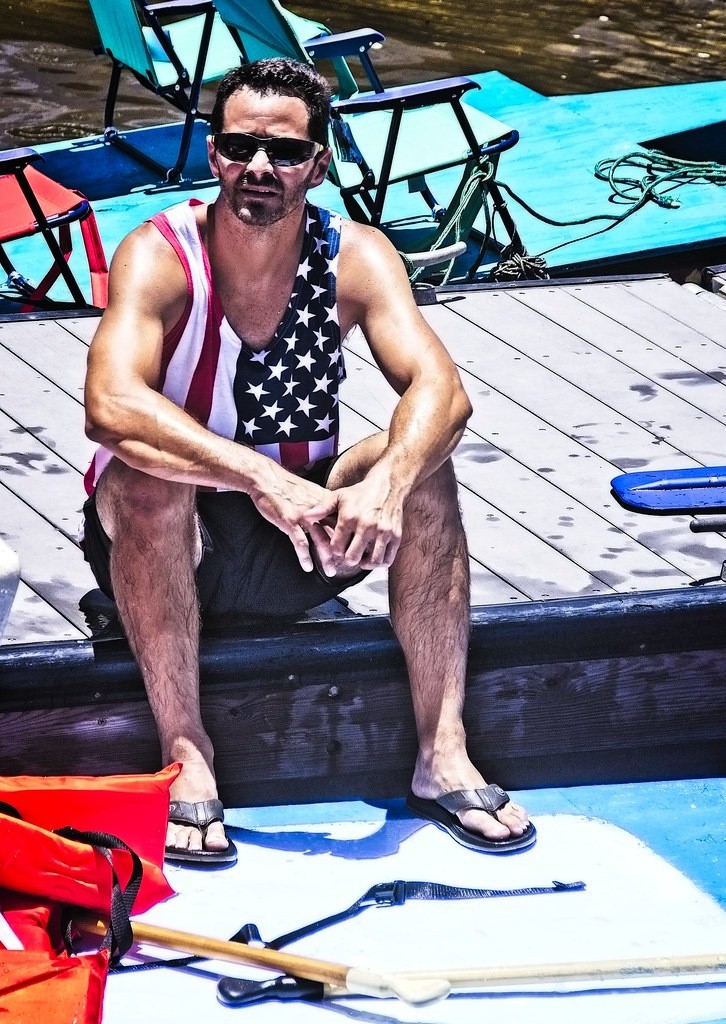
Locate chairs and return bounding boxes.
[0,148,109,310]
[88,0,528,282]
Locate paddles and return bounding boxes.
[610,464,726,513]
[72,903,455,1006]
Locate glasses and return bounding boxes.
[206,132,324,167]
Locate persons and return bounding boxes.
[78,55,537,869]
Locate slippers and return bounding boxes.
[405,783,538,857]
[161,798,239,862]
